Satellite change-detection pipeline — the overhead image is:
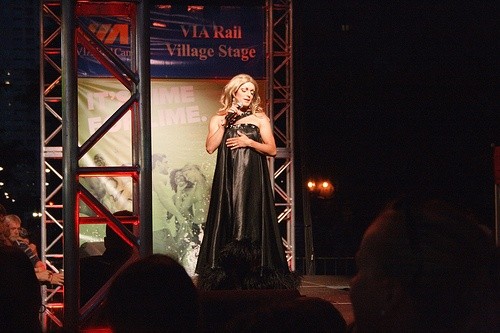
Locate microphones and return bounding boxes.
[225,101,243,121]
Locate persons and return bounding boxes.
[79,209,134,310]
[194,74,303,293]
[222,296,349,333]
[2,214,66,287]
[0,245,44,333]
[0,204,8,245]
[347,186,498,333]
[105,253,200,333]
[17,227,40,259]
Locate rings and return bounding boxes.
[232,144,234,147]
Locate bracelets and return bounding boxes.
[221,119,226,127]
